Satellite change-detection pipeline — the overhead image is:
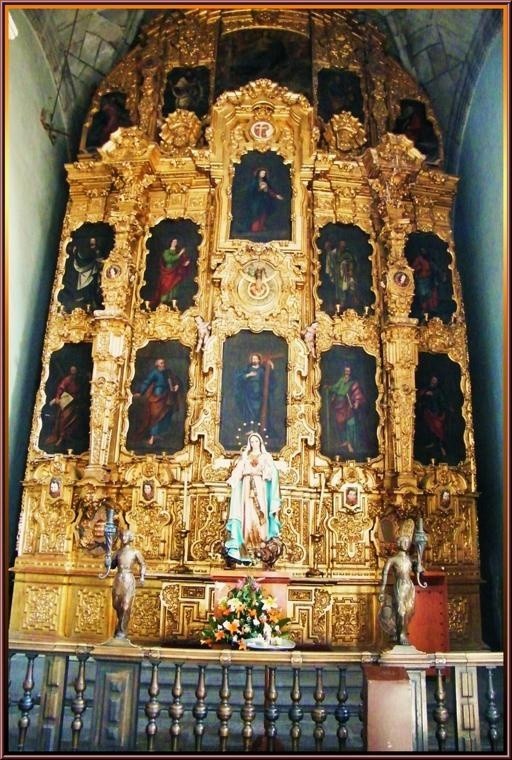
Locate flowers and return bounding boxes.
[198,574,285,651]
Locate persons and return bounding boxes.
[438,491,451,510]
[169,67,206,115]
[344,488,359,509]
[328,72,361,119]
[142,484,156,500]
[405,244,448,327]
[228,350,278,444]
[67,235,106,316]
[47,481,62,498]
[316,360,364,453]
[94,99,125,148]
[130,357,182,447]
[222,432,281,566]
[41,364,89,450]
[239,166,287,235]
[319,237,363,316]
[399,102,421,143]
[104,528,146,640]
[377,534,428,647]
[240,26,282,73]
[412,368,457,465]
[147,237,189,314]
